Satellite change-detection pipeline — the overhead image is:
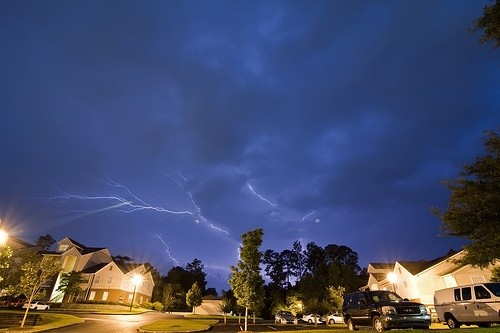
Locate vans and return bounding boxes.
[434,282,500,328]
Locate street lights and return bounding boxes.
[130,274,143,311]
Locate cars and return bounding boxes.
[22,300,50,311]
[0,296,14,308]
[275,311,298,324]
[7,298,26,309]
[327,313,346,324]
[302,312,325,324]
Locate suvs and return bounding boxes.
[342,290,431,333]
